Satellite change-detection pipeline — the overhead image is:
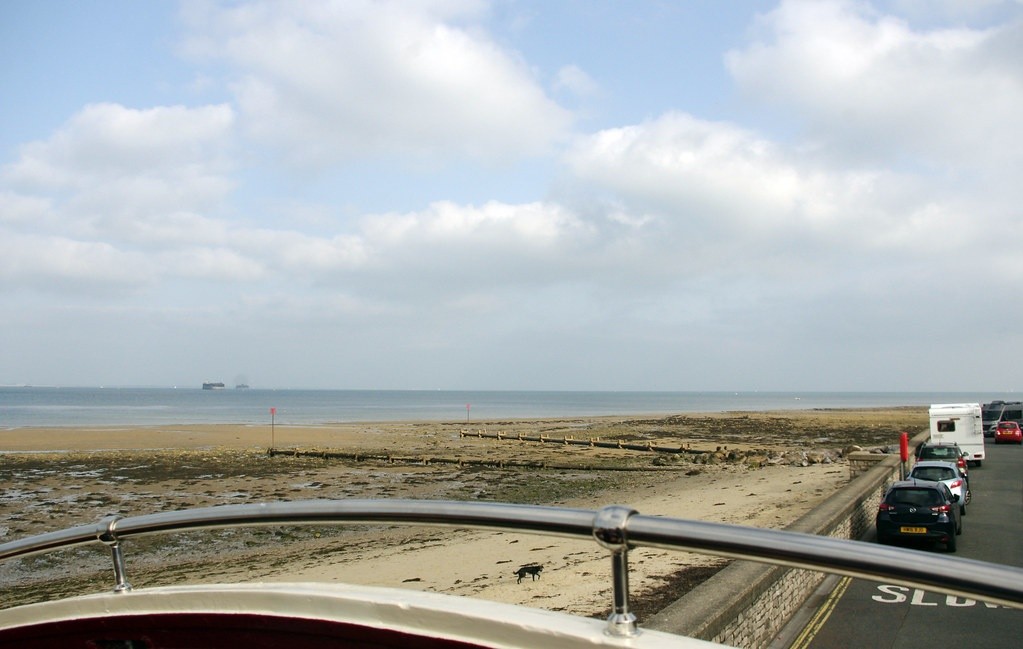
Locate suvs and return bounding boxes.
[917,441,969,489]
[906,460,968,516]
[876,480,962,554]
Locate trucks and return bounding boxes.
[928,403,985,467]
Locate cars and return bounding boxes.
[995,422,1023,444]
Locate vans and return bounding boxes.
[982,400,1023,437]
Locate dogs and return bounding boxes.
[512,565,545,584]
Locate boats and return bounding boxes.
[203,383,226,390]
